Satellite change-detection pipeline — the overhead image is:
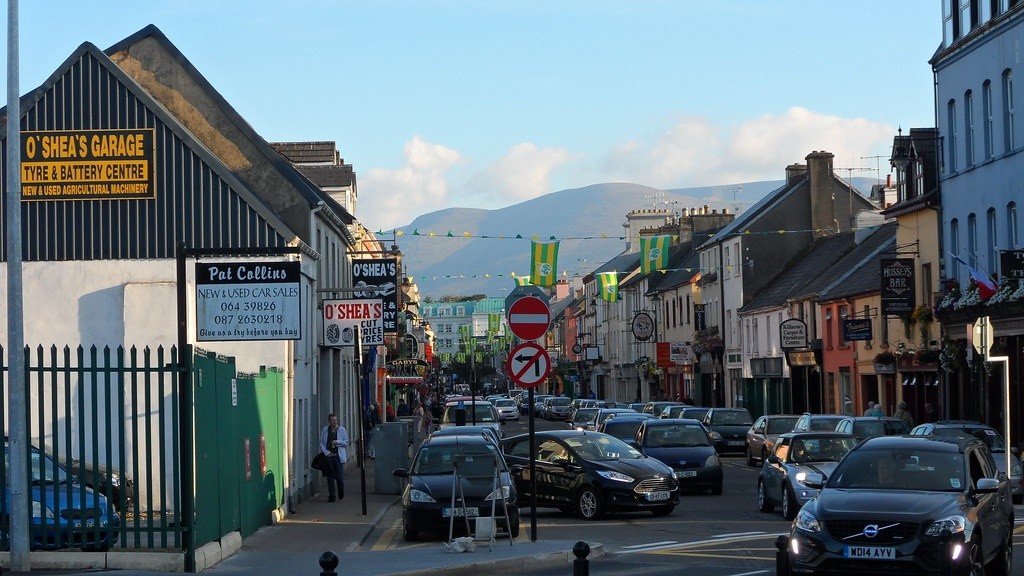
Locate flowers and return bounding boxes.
[933,273,1024,311]
[900,303,933,350]
[647,362,663,376]
[691,331,721,353]
[895,336,993,379]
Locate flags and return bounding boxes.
[514,276,535,287]
[529,241,560,287]
[433,314,517,364]
[597,273,619,303]
[955,256,997,300]
[640,236,670,275]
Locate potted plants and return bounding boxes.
[872,349,896,374]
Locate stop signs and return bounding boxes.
[508,297,550,340]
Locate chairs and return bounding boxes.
[422,452,445,474]
[683,431,701,443]
[651,430,667,444]
[713,414,724,423]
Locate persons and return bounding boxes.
[894,401,914,431]
[864,401,885,417]
[633,399,640,403]
[555,389,596,399]
[683,395,695,406]
[319,414,349,501]
[924,404,939,422]
[367,389,512,454]
[650,393,669,402]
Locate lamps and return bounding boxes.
[643,286,678,301]
[887,125,945,175]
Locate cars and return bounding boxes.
[391,382,524,541]
[499,430,678,520]
[0,435,133,551]
[788,436,1013,576]
[507,389,802,495]
[758,431,876,520]
[905,419,1022,502]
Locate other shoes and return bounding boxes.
[338,492,344,499]
[328,496,335,502]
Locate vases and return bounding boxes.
[934,293,1024,325]
[896,354,939,372]
[652,375,659,379]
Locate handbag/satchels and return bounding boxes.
[311,450,328,477]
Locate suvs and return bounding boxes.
[792,412,910,468]
